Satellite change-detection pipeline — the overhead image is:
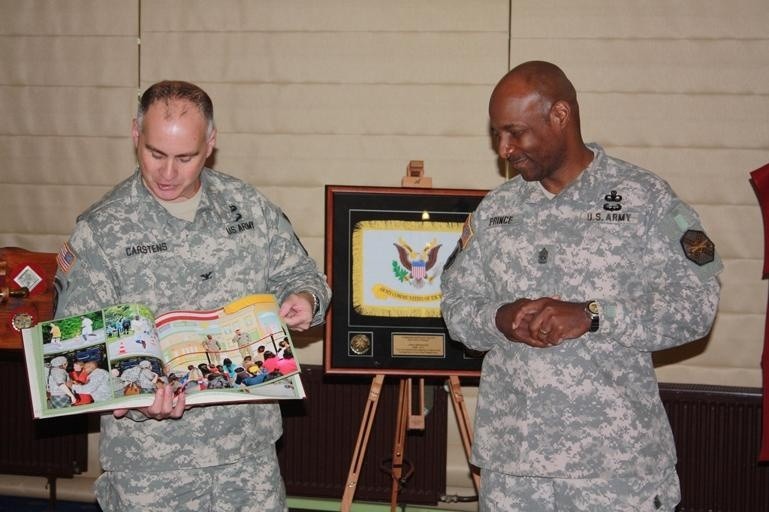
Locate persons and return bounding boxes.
[438,59,724,512]
[80,316,97,342]
[46,355,167,409]
[202,335,222,364]
[49,80,333,512]
[106,313,143,338]
[50,322,62,347]
[231,328,252,359]
[166,337,298,399]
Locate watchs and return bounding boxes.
[583,301,602,334]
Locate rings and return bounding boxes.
[540,328,549,335]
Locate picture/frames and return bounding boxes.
[329,188,498,374]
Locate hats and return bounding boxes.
[139,360,151,368]
[51,356,67,366]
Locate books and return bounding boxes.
[20,292,307,419]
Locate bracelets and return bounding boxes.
[308,291,319,317]
